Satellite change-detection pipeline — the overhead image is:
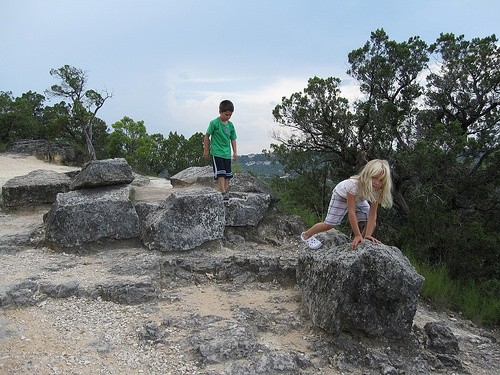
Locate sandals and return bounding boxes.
[300,232,322,249]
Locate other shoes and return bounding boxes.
[222,192,229,200]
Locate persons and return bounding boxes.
[300,159,393,250]
[203,100,238,200]
